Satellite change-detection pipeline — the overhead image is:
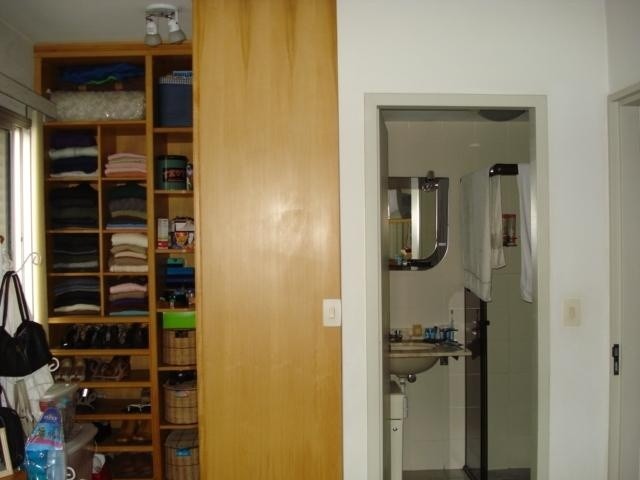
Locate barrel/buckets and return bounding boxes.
[155,154,187,190]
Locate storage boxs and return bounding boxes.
[157,79,193,128]
[39,380,83,442]
[62,422,100,478]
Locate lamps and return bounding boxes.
[143,3,188,47]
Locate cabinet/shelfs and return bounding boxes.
[145,40,199,479]
[30,40,160,479]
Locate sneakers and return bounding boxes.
[79,388,96,411]
[54,356,130,383]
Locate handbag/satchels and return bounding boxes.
[0,268,52,377]
[1,380,34,469]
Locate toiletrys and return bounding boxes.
[424,326,454,342]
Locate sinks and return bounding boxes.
[388,337,473,378]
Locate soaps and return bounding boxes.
[410,336,424,341]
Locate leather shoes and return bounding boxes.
[117,420,152,443]
[113,453,153,478]
[61,324,148,349]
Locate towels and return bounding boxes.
[514,162,538,304]
[459,165,508,304]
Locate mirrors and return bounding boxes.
[386,169,450,272]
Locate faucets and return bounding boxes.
[389,329,402,342]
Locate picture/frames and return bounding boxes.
[0,426,14,479]
